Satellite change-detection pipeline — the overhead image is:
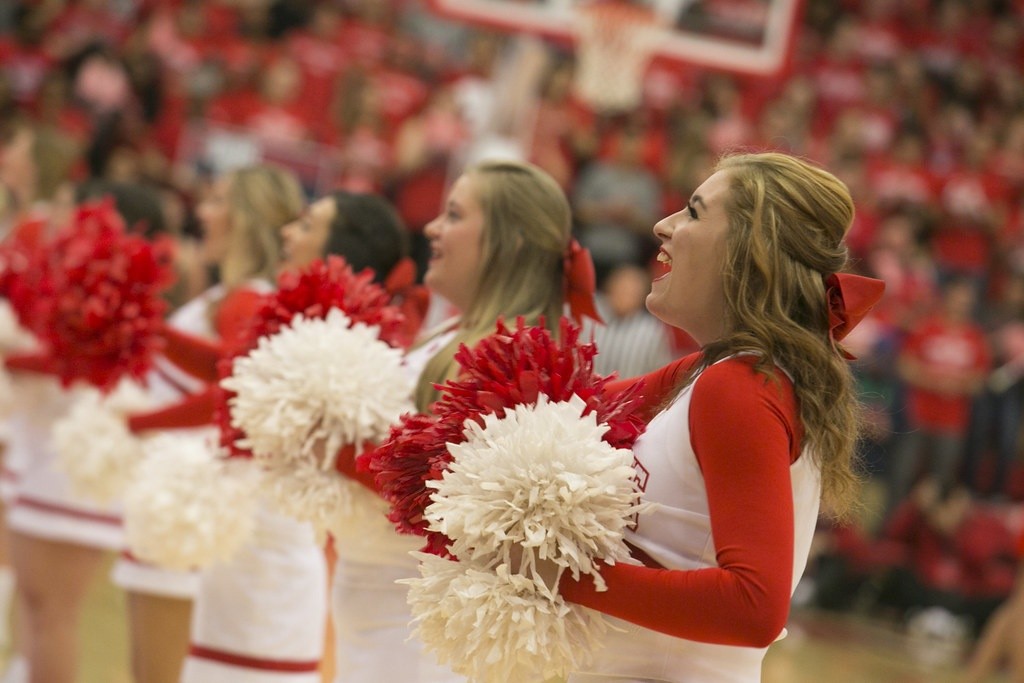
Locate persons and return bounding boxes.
[0,0,1024,683]
[421,151,885,683]
[327,161,606,683]
